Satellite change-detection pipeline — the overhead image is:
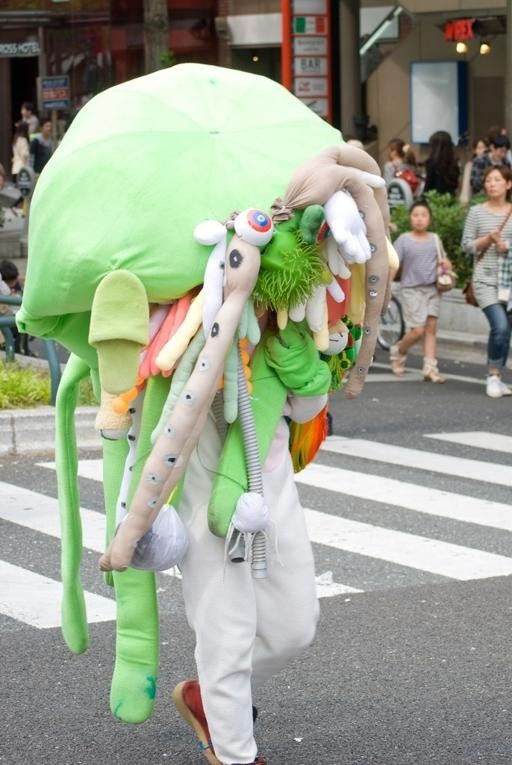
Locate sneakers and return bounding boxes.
[171,678,221,764]
[486,374,511,397]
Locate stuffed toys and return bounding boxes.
[16,61,400,765]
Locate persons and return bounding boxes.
[459,165,512,398]
[390,201,457,382]
[2,102,52,356]
[384,125,511,209]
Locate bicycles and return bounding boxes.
[375,294,406,352]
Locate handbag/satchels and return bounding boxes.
[436,263,453,293]
[394,168,417,192]
[463,281,478,307]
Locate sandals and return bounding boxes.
[423,358,446,384]
[390,344,406,375]
